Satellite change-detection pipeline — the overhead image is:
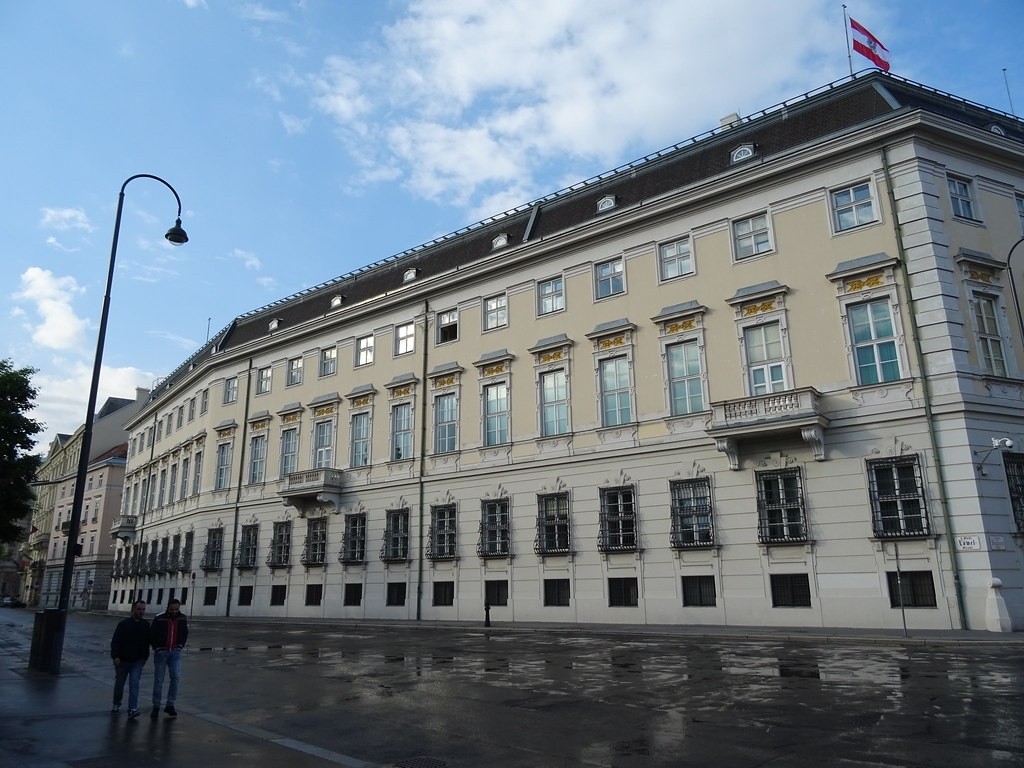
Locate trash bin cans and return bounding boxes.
[28,608,58,676]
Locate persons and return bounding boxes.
[150,599,188,718]
[111,600,151,718]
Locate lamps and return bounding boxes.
[992,438,1014,449]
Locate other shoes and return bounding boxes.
[111,705,121,716]
[164,706,178,716]
[128,708,140,719]
[150,709,159,720]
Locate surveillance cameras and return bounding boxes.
[1005,440,1014,448]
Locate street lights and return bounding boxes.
[50,174,189,673]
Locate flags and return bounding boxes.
[849,17,892,74]
[19,553,32,567]
[32,526,38,533]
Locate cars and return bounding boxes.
[2,598,12,606]
